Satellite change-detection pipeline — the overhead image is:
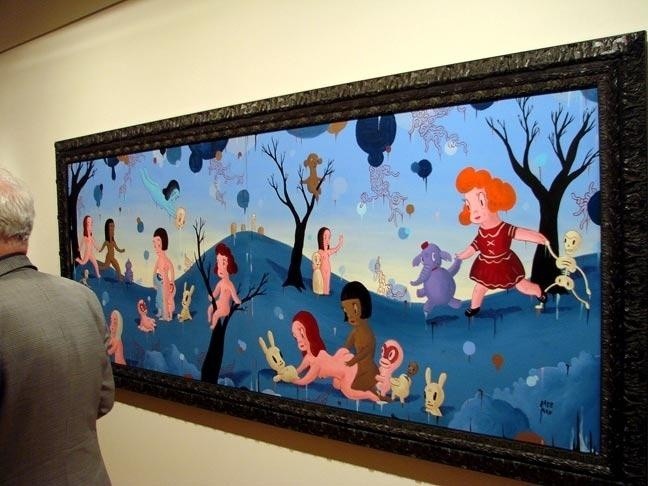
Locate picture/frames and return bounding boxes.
[55,30,648,486]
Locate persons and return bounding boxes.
[2,167,115,481]
